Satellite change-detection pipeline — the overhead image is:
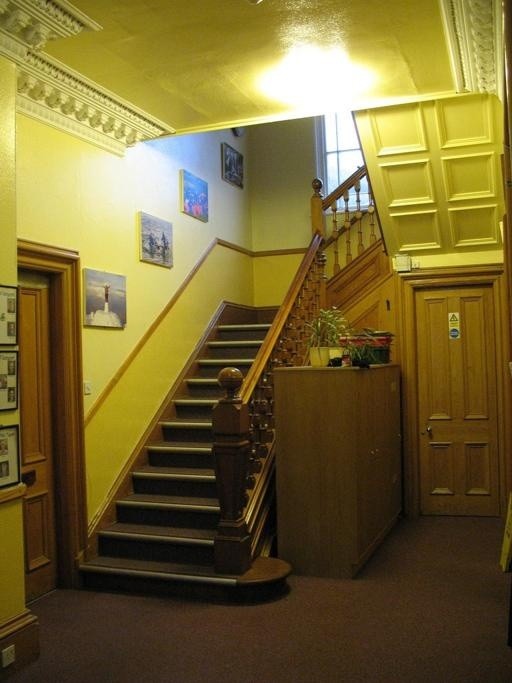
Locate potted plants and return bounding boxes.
[301,304,385,368]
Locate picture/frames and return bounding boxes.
[0,282,22,489]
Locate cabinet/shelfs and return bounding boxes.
[270,362,404,580]
